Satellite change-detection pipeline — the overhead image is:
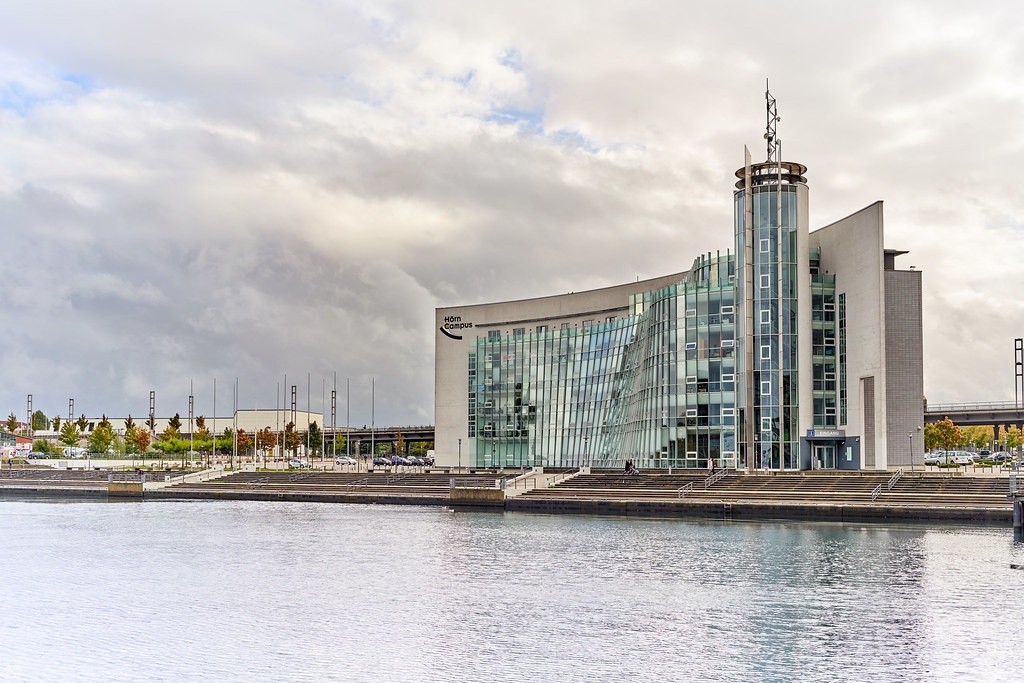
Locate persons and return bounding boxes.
[708,458,716,475]
[623,459,633,475]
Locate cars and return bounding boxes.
[335,456,357,465]
[28,451,50,459]
[289,459,311,470]
[372,455,432,467]
[924,449,1013,466]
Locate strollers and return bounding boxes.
[629,466,640,474]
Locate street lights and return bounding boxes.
[754,434,760,476]
[492,440,496,472]
[583,437,590,462]
[909,435,914,475]
[458,438,462,474]
[394,441,399,474]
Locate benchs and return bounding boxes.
[470,469,497,474]
[367,468,391,473]
[94,466,113,471]
[424,469,450,474]
[66,466,84,470]
